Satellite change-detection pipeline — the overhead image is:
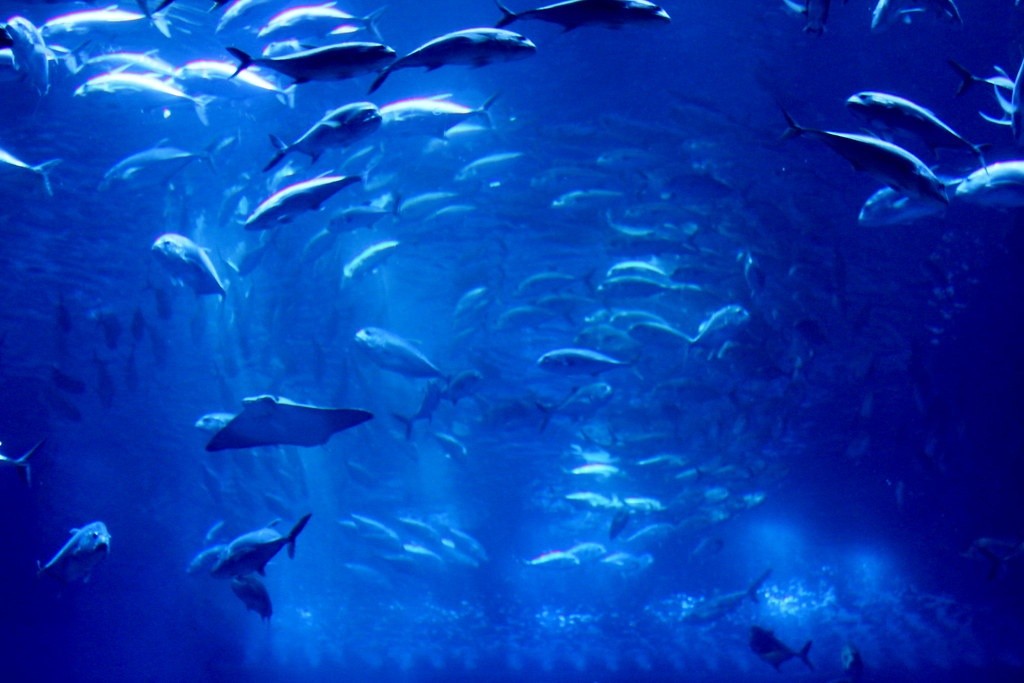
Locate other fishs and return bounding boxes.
[0,1,1024,683]
